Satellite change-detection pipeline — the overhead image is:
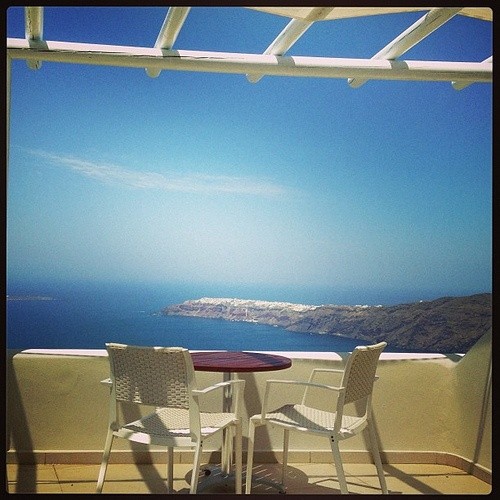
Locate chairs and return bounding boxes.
[245,342,388,494]
[95,342,236,494]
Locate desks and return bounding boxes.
[188,351,291,495]
